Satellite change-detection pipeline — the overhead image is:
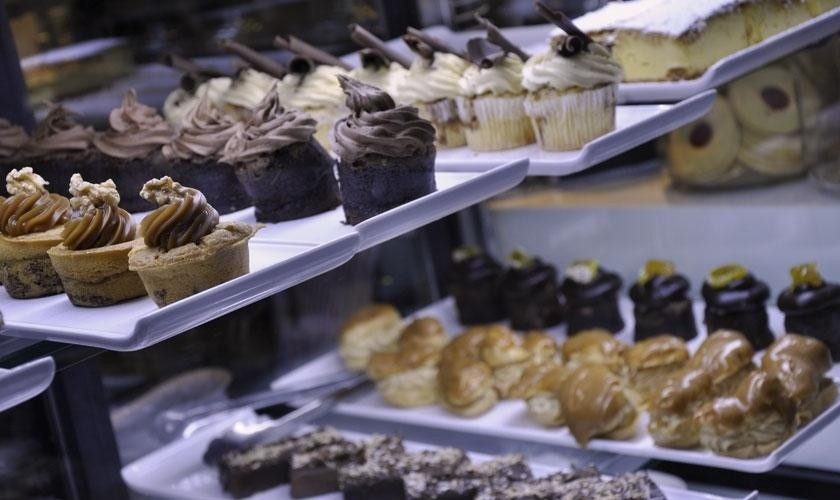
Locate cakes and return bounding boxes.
[160,52,233,122]
[213,423,345,499]
[329,74,437,226]
[47,172,147,307]
[266,34,345,140]
[522,0,616,153]
[505,472,667,500]
[498,248,566,329]
[391,27,465,151]
[216,82,341,223]
[454,13,535,153]
[0,166,66,298]
[628,261,697,344]
[159,91,253,216]
[127,175,256,310]
[557,261,625,336]
[447,245,504,324]
[472,464,602,500]
[0,118,32,185]
[698,264,776,350]
[0,115,29,194]
[333,447,472,500]
[777,264,840,368]
[332,21,410,139]
[402,455,531,500]
[286,434,406,499]
[215,37,279,118]
[3,104,98,197]
[92,87,176,213]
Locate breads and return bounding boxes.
[337,304,403,371]
[544,1,839,83]
[525,328,627,435]
[557,334,691,450]
[689,332,837,460]
[643,330,757,451]
[369,316,443,407]
[437,326,555,417]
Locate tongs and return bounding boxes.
[149,369,374,465]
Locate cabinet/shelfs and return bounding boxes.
[0,2,840,499]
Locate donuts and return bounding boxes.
[664,39,836,191]
